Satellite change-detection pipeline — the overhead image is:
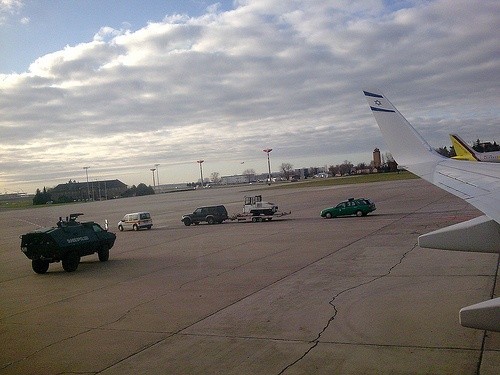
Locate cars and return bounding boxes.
[320,197,377,219]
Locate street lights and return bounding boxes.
[82,166,91,200]
[150,169,156,183]
[197,160,205,187]
[263,147,272,180]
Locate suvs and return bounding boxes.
[180,204,229,226]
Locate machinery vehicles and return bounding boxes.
[19,212,116,274]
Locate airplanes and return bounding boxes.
[363,90,500,333]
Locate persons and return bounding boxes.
[104,218,108,230]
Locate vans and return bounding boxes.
[118,212,153,231]
